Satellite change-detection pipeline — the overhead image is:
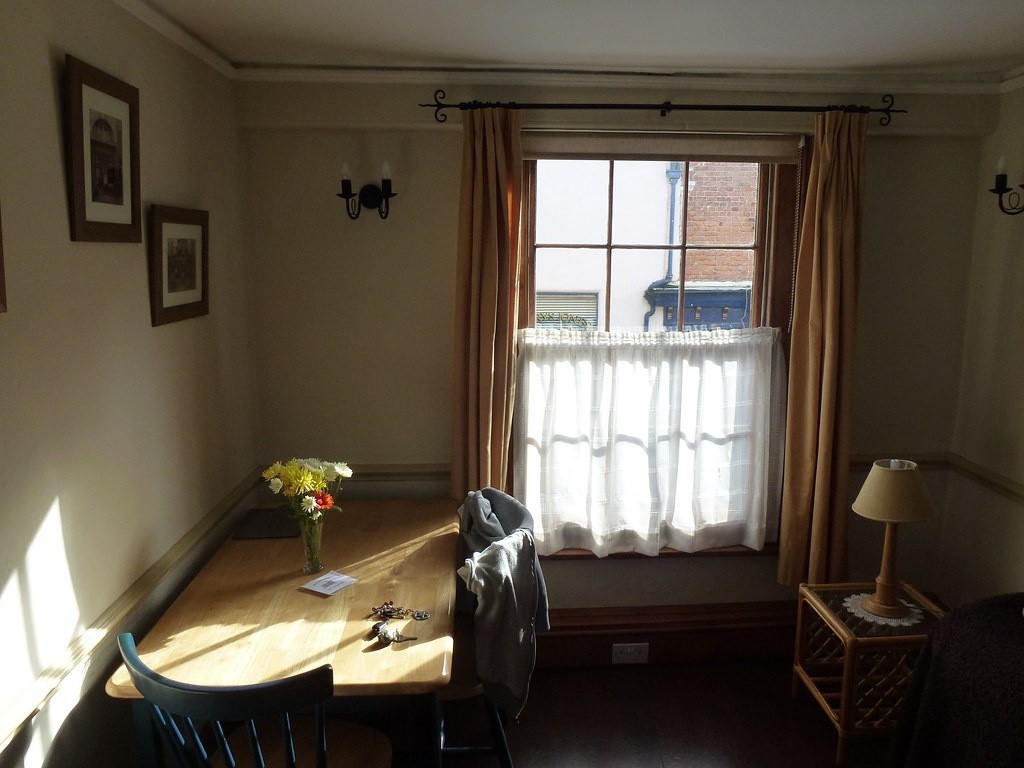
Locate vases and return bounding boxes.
[300,520,325,574]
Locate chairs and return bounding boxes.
[116,633,393,768]
[376,487,550,767]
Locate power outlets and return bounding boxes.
[613,643,649,664]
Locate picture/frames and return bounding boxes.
[64,56,143,243]
[151,205,210,328]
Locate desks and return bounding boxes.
[106,502,460,768]
[795,581,944,768]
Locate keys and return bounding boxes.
[372,601,432,642]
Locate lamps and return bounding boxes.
[989,155,1024,214]
[851,458,936,619]
[338,177,395,220]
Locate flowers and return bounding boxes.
[262,458,354,563]
